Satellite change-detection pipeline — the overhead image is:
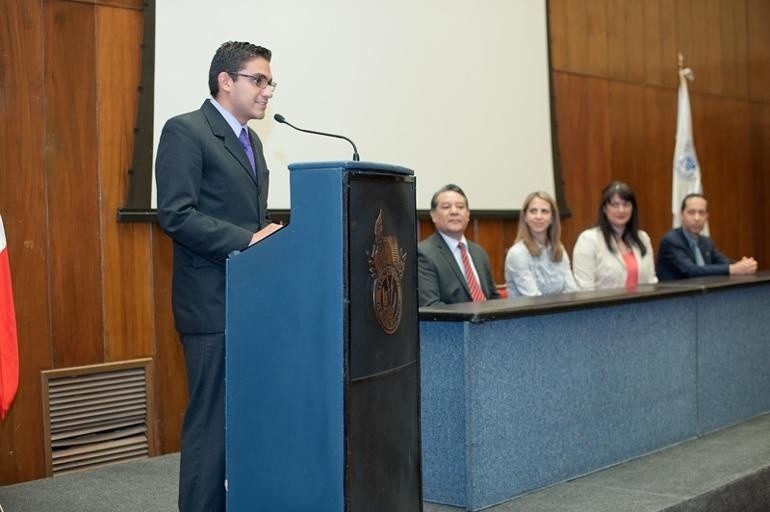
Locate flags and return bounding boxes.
[671,68,711,240]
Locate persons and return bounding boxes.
[155,40,287,512]
[504,190,581,298]
[571,181,659,292]
[656,194,758,284]
[418,182,499,307]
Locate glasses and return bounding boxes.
[229,69,276,92]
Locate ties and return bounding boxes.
[457,241,486,303]
[693,241,706,266]
[239,128,256,181]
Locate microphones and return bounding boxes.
[274,113,359,162]
